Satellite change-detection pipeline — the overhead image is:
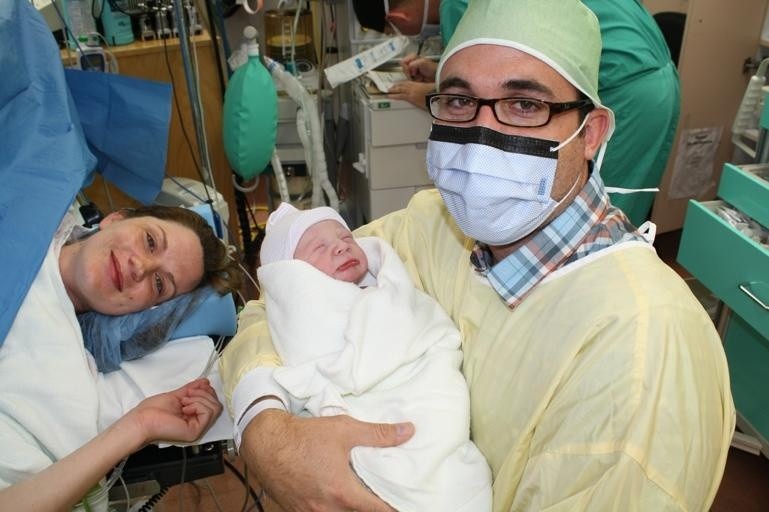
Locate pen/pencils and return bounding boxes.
[411,40,425,78]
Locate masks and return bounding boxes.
[402,23,442,44]
[427,111,592,248]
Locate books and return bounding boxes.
[364,69,408,94]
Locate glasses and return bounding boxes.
[425,94,592,128]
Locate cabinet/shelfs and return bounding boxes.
[640,0,769,237]
[59,25,271,310]
[348,75,435,232]
[676,88,768,462]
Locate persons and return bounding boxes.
[214,1,734,510]
[259,202,494,509]
[0,199,230,511]
[349,0,682,236]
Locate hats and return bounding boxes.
[255,204,355,270]
[435,0,609,109]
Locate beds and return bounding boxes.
[76,312,237,489]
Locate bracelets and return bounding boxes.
[233,393,290,457]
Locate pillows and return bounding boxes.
[166,203,237,340]
